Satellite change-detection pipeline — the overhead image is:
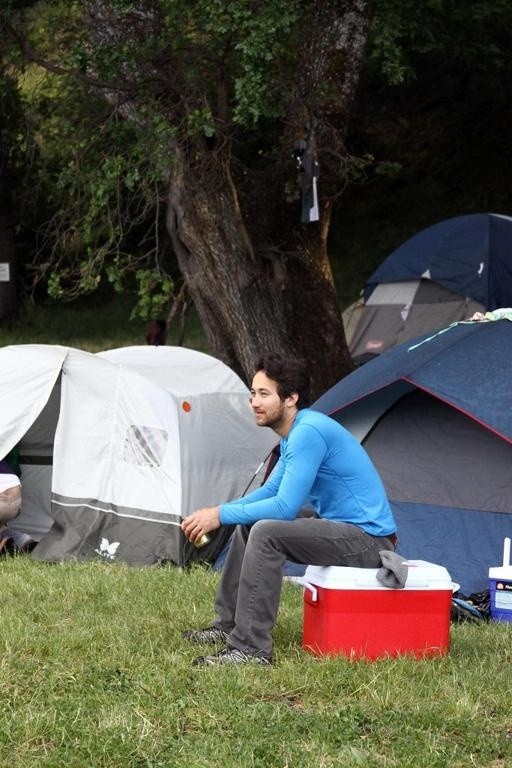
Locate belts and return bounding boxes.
[388,534,398,546]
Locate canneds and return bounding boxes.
[193,532,212,548]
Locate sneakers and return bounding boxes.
[181,627,271,671]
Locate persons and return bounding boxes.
[181,352,399,666]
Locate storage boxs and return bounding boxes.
[292,558,460,661]
